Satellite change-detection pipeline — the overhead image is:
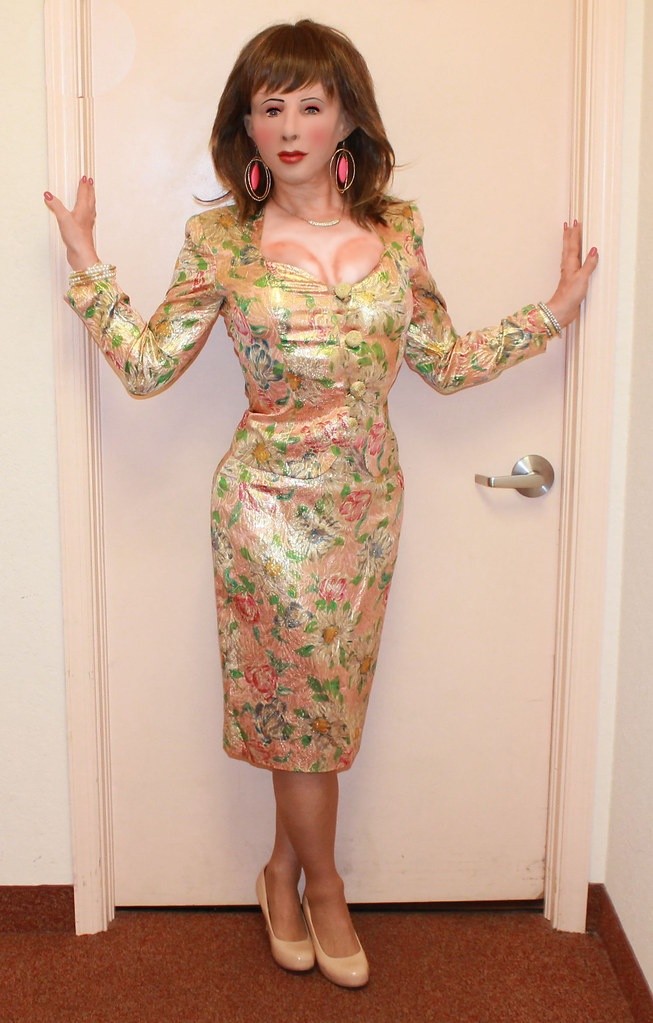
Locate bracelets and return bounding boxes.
[68,263,116,286]
[536,302,561,338]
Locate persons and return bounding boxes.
[43,21,599,989]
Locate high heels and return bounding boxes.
[256,861,315,971]
[302,891,368,987]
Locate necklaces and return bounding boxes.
[269,195,344,227]
